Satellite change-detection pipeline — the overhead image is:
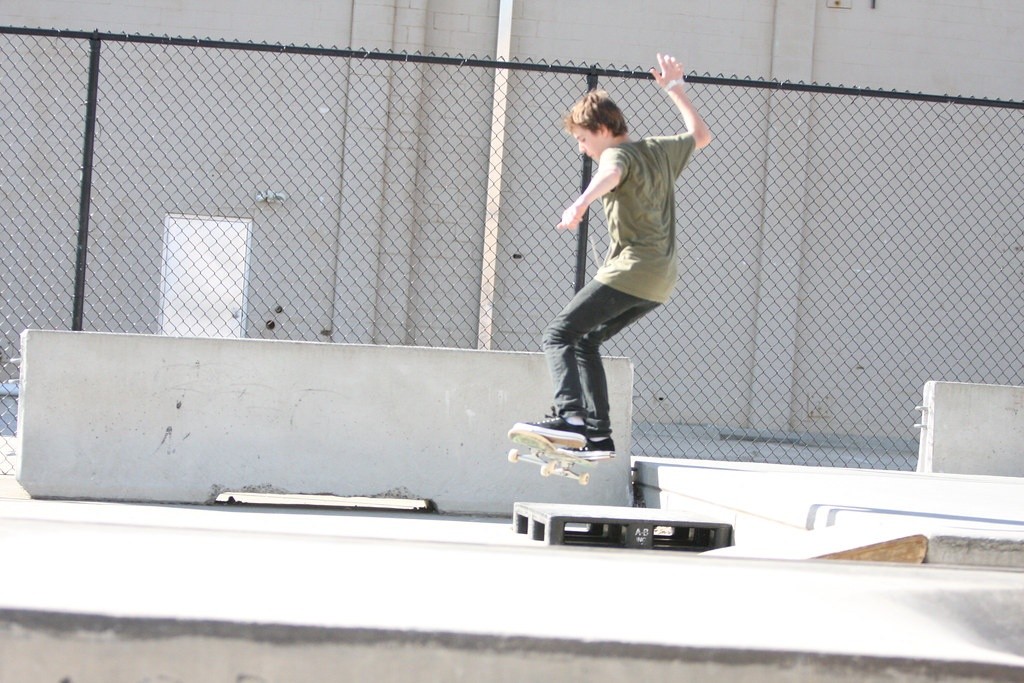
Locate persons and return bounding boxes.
[510,51,713,464]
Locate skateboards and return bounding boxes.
[507,429,597,485]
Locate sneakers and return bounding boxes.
[555,436,617,461]
[513,406,589,451]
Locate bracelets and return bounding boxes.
[665,78,684,93]
